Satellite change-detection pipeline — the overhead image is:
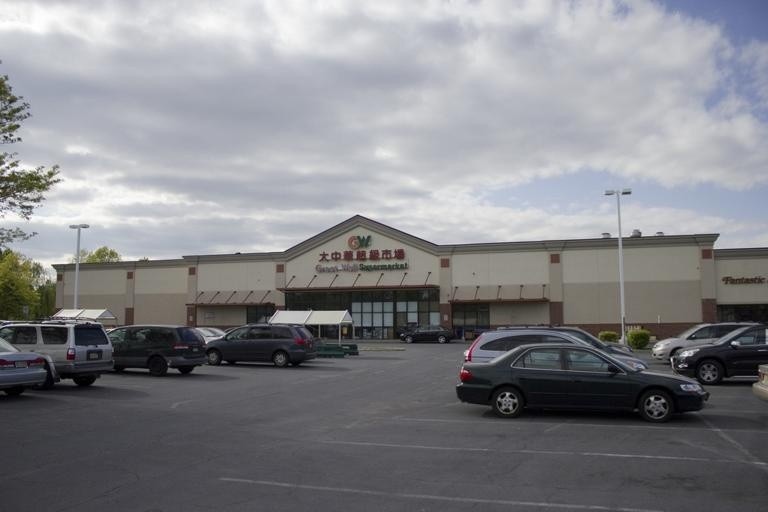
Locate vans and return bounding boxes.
[0,317,114,391]
[205,322,317,368]
[106,325,208,377]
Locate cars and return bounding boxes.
[497,323,633,359]
[464,329,649,371]
[191,327,227,344]
[602,340,633,352]
[670,324,768,385]
[652,321,762,369]
[456,343,711,423]
[0,336,47,397]
[752,363,768,402]
[400,325,454,344]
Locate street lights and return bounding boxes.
[69,224,89,309]
[605,187,632,347]
